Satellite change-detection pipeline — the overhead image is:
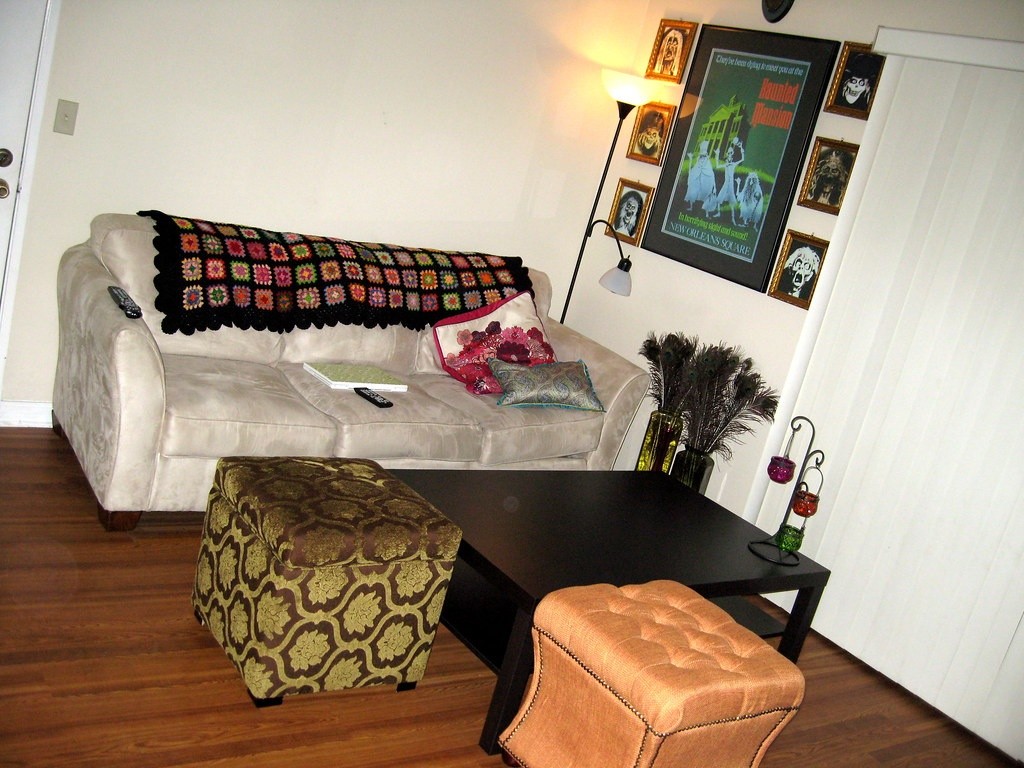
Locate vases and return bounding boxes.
[634,404,683,475]
[670,444,715,496]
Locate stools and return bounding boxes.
[497,580,806,768]
[189,455,462,708]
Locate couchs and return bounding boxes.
[52,209,650,533]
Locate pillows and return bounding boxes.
[488,356,606,413]
[432,292,558,396]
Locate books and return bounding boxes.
[302,362,408,393]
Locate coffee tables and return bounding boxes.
[386,468,833,756]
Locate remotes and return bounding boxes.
[107,286,143,319]
[354,387,393,408]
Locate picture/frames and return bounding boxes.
[603,177,655,248]
[646,19,700,84]
[640,23,841,295]
[796,136,860,216]
[821,40,886,121]
[626,102,678,167]
[767,229,829,310]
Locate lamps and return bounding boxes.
[559,81,649,324]
